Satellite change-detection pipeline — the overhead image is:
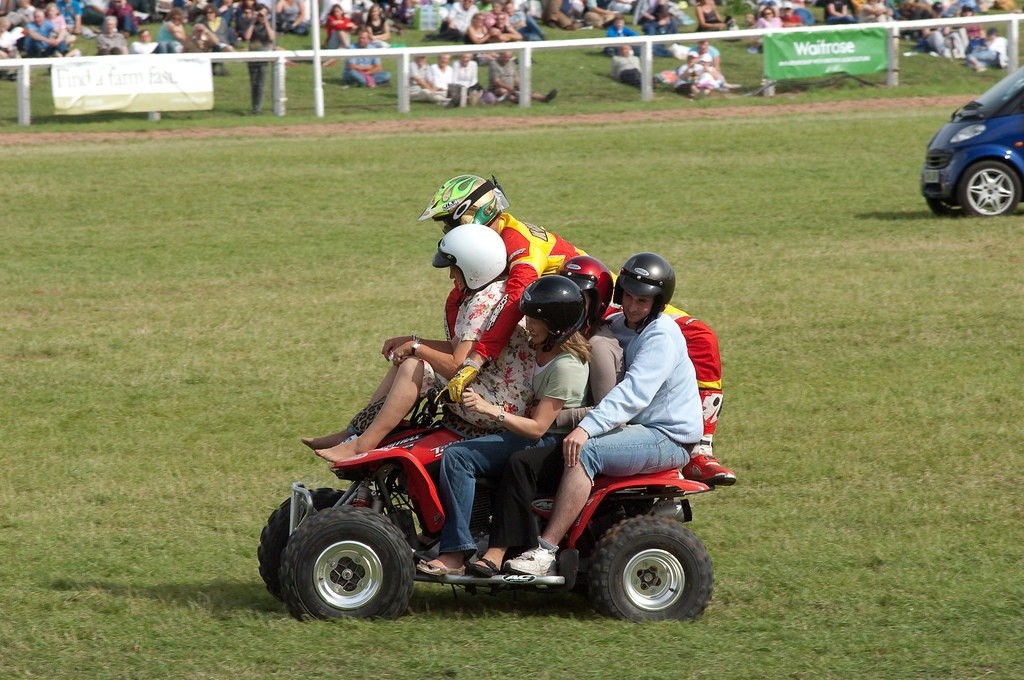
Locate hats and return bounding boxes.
[650,5,674,17]
[932,2,942,13]
[687,51,713,64]
[782,2,791,9]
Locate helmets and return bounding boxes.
[614,253,676,320]
[559,257,614,320]
[415,176,502,229]
[431,224,507,290]
[519,276,588,347]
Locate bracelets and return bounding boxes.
[463,357,481,371]
[411,342,423,357]
[411,335,416,342]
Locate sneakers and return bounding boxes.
[503,547,557,577]
[683,455,736,485]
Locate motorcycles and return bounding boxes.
[257,386,715,625]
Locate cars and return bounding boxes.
[920,65,1024,217]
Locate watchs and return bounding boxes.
[497,406,505,423]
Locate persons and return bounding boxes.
[0,0,1024,115]
[301,176,736,576]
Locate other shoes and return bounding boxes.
[471,559,500,576]
[415,560,466,576]
[544,89,557,104]
